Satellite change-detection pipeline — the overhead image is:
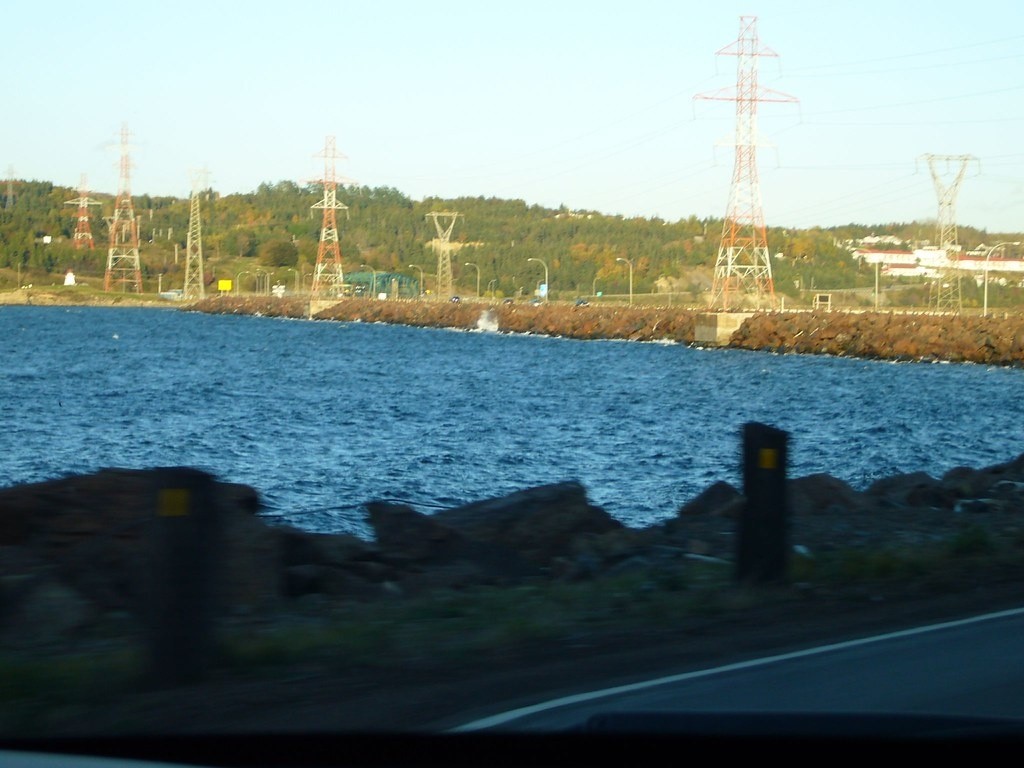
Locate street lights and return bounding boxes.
[465,262,480,297]
[360,264,376,298]
[617,257,633,306]
[527,257,548,302]
[236,270,249,297]
[983,241,1020,317]
[302,273,313,292]
[263,271,274,294]
[288,268,300,291]
[408,265,423,296]
[256,268,270,295]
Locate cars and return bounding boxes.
[503,298,516,306]
[575,299,591,308]
[527,298,544,308]
[448,297,460,304]
